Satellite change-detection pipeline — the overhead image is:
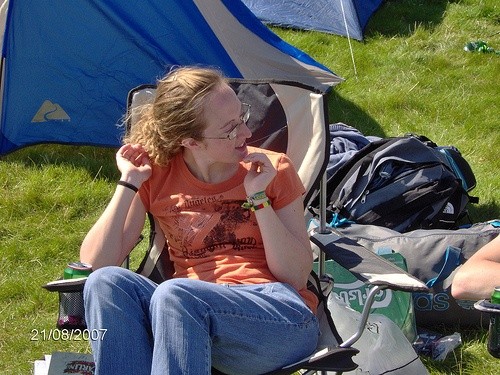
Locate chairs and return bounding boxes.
[41,79,428,375]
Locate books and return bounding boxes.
[35,351,95,375]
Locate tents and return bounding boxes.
[241,0,385,44]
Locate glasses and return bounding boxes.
[197,102,251,140]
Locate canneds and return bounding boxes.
[63,261,93,315]
[488,286,500,358]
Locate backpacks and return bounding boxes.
[326,134,477,231]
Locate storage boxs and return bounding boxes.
[311,248,418,343]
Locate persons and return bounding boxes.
[451,232,500,304]
[81,65,321,375]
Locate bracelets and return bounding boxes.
[116,181,139,193]
[241,191,272,212]
[0,0,346,154]
